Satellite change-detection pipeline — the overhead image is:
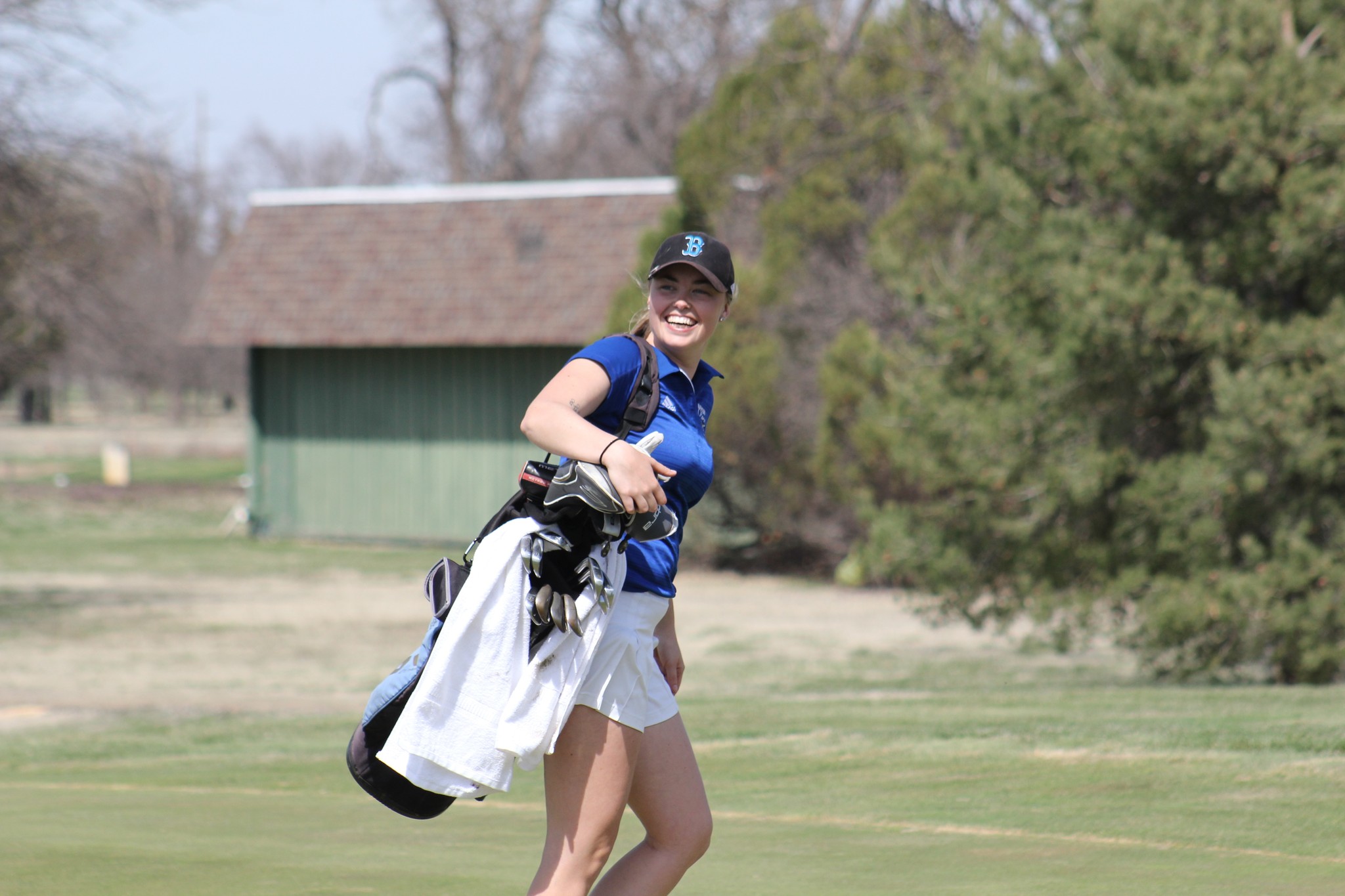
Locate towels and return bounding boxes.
[372,518,628,799]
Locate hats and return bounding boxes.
[647,231,735,305]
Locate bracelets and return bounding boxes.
[599,438,623,468]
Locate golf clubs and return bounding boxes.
[519,528,615,662]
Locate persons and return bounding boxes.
[522,231,736,896]
